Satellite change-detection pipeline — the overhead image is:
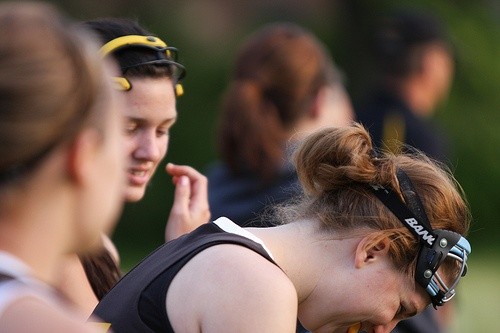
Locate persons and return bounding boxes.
[1,0,472,333]
